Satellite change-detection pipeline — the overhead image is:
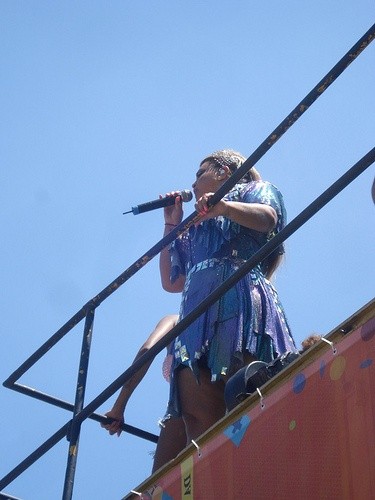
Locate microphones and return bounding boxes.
[123,189,193,215]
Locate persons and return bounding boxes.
[99,313,230,477]
[161,148,300,447]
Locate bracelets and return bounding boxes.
[165,223,177,227]
